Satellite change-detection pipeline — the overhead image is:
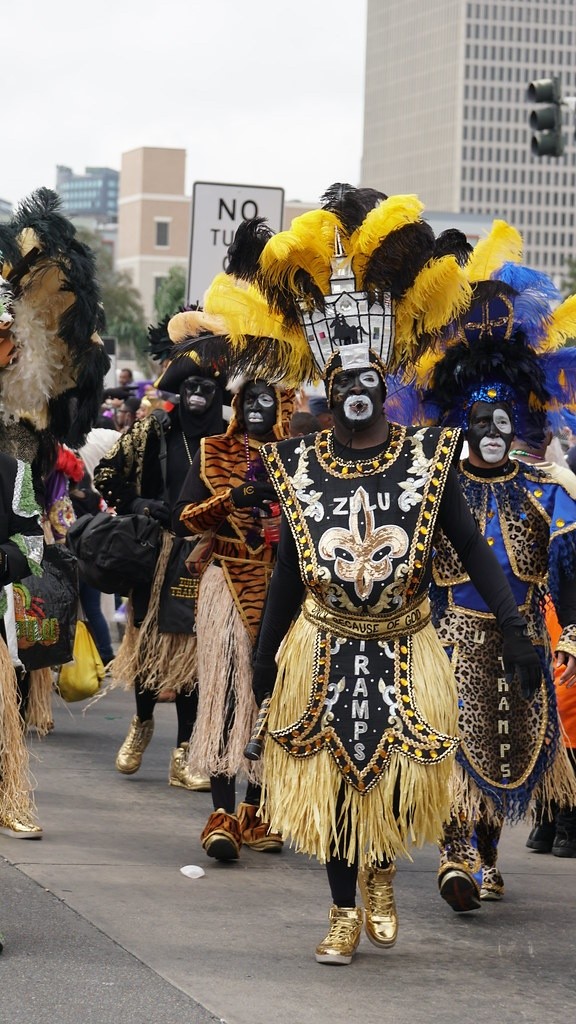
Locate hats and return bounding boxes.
[167,271,319,440]
[145,301,251,394]
[385,220,575,447]
[203,184,473,409]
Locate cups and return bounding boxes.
[180,865,205,878]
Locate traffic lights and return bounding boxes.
[525,76,565,157]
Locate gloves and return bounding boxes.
[231,480,279,513]
[127,498,168,522]
[251,654,279,707]
[501,618,543,700]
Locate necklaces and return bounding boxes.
[181,427,193,465]
[243,431,252,483]
[509,449,546,460]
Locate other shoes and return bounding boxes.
[248,841,282,852]
[479,888,501,899]
[440,870,480,912]
[204,834,238,860]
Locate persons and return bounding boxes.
[145,181,576,963]
[0,185,285,863]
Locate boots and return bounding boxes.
[552,799,576,857]
[526,798,555,850]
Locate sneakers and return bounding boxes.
[115,715,154,774]
[316,904,362,964]
[358,860,398,948]
[0,818,42,838]
[169,742,211,790]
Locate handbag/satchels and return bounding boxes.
[49,602,104,703]
[79,512,162,582]
